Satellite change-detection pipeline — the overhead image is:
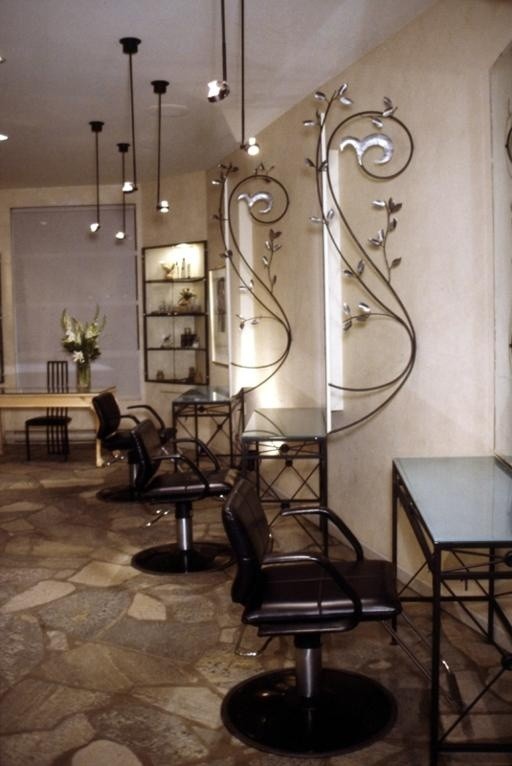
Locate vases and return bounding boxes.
[77,363,90,391]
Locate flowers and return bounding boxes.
[61,303,106,381]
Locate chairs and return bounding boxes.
[25,361,72,462]
[92,392,239,574]
[221,479,398,759]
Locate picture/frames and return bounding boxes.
[209,266,231,368]
[142,241,208,387]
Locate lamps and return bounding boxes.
[0,57,8,141]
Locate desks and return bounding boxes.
[390,457,512,766]
[173,385,328,556]
[0,386,122,468]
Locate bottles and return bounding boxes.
[175,261,179,278]
[181,258,186,278]
[187,263,190,278]
